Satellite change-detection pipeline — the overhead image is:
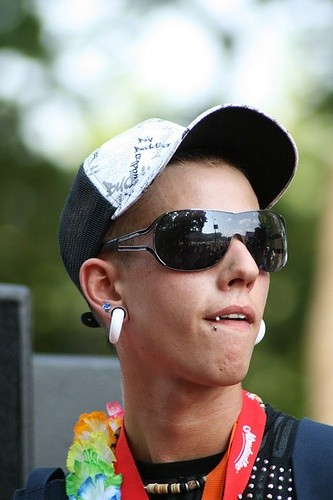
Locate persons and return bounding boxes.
[11,102,333,500]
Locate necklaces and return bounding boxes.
[65,389,266,500]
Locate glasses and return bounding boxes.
[103,209,289,273]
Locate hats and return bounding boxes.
[58,103,300,328]
